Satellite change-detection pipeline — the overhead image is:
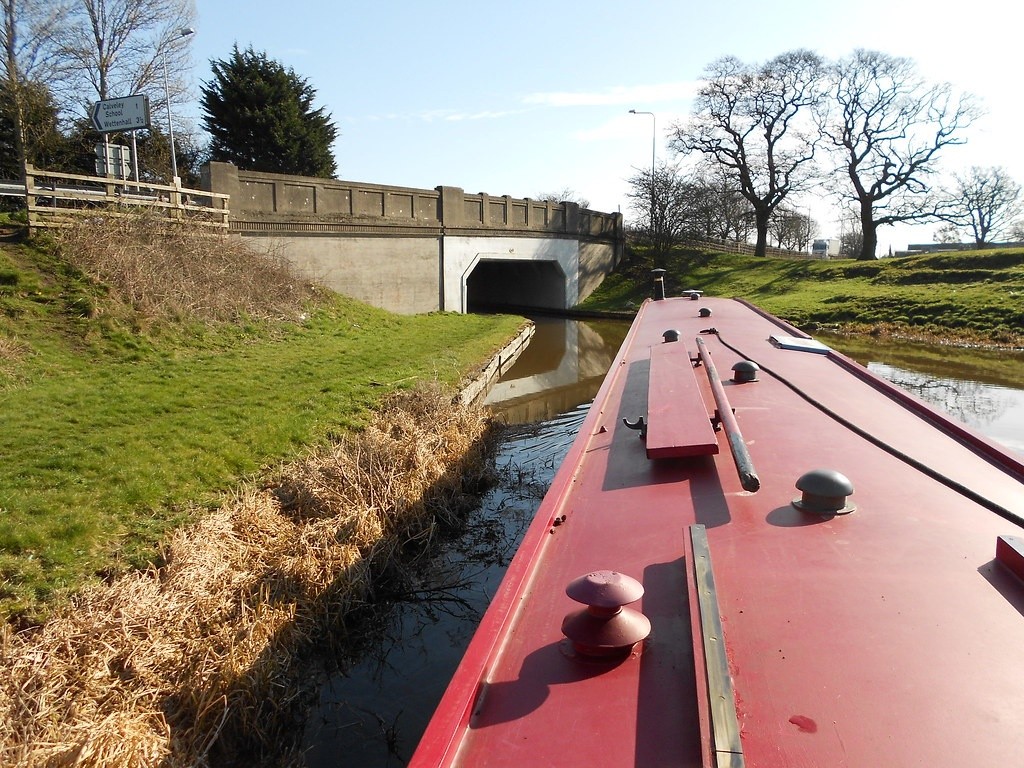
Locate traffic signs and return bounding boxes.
[91,94,150,133]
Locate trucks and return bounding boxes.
[812,238,841,261]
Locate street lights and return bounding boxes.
[162,29,193,221]
[629,110,655,232]
